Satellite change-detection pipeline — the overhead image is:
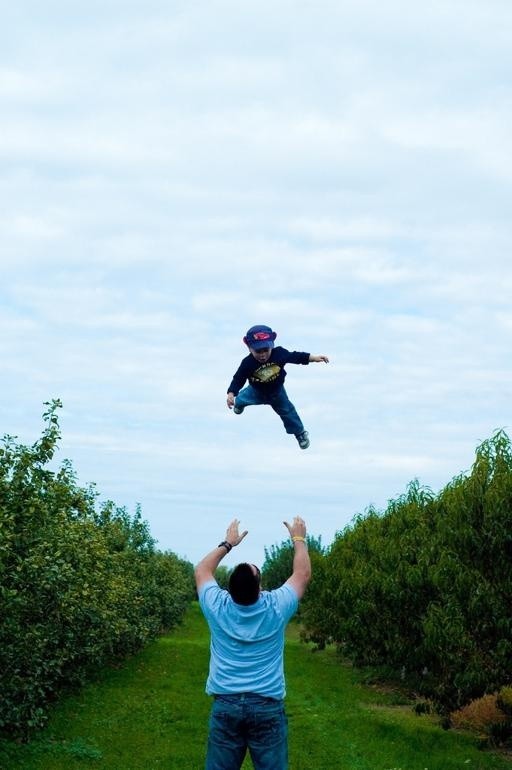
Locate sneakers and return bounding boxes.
[295,430,310,450]
[232,405,245,415]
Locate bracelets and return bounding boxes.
[220,541,233,553]
[290,536,307,544]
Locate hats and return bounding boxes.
[244,324,275,350]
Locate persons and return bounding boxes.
[224,324,329,451]
[194,515,313,769]
